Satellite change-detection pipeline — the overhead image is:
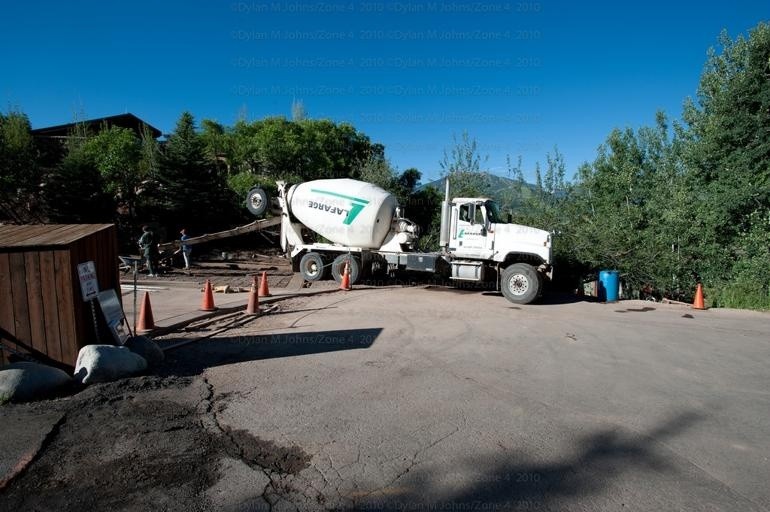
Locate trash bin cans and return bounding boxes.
[600,271,619,301]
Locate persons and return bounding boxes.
[180,228,193,270]
[139,225,161,274]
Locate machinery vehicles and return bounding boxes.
[275,177,555,305]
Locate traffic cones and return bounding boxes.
[340,259,352,291]
[246,271,272,316]
[692,284,706,310]
[200,279,218,310]
[137,291,157,332]
